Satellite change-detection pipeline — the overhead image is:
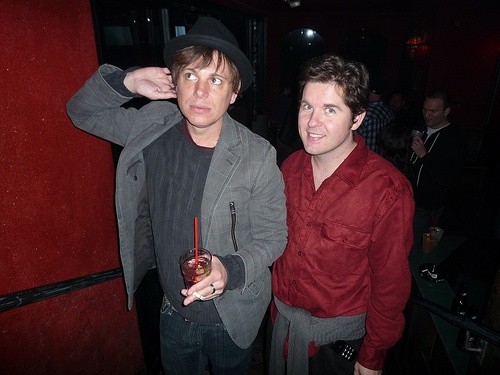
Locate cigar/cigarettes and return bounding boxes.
[192,292,206,302]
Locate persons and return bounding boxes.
[65,16,288,375]
[356,85,396,153]
[401,84,478,279]
[259,56,416,375]
[376,117,417,176]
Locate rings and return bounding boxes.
[210,284,216,294]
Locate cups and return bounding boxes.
[412,129,423,142]
[422,233,439,253]
[429,227,444,241]
[179,248,212,290]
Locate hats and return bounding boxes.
[165,17,252,92]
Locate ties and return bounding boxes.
[410,132,428,164]
